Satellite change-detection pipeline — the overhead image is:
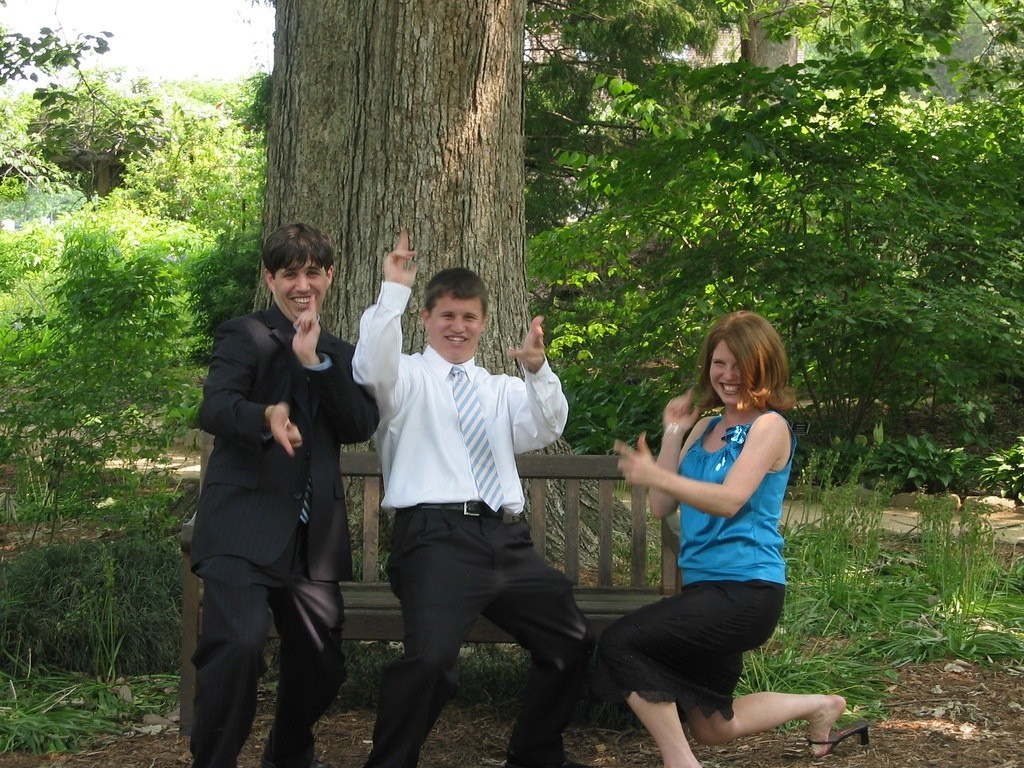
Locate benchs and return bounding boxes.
[172,434,681,748]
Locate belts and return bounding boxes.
[417,501,504,516]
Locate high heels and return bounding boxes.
[806,718,870,759]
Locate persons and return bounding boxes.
[599,310,869,768]
[190,223,381,768]
[351,231,591,767]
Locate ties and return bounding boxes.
[299,475,312,524]
[450,363,504,513]
